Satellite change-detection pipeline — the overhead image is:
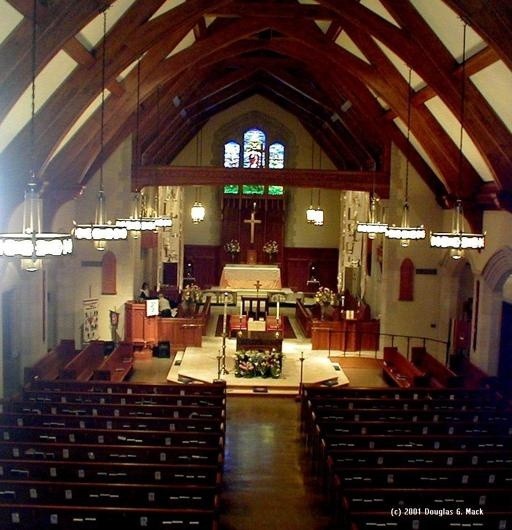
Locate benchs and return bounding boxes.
[377,346,462,390]
[298,384,512,529]
[0,380,227,530]
[24,339,134,383]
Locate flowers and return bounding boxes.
[223,237,241,255]
[262,239,280,256]
[233,349,286,378]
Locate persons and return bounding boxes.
[139,282,150,299]
[158,293,171,318]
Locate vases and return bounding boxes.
[231,253,235,264]
[268,254,272,264]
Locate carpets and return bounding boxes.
[215,313,297,339]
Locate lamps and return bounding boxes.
[355,20,486,260]
[306,139,325,227]
[2,2,174,261]
[190,128,206,225]
